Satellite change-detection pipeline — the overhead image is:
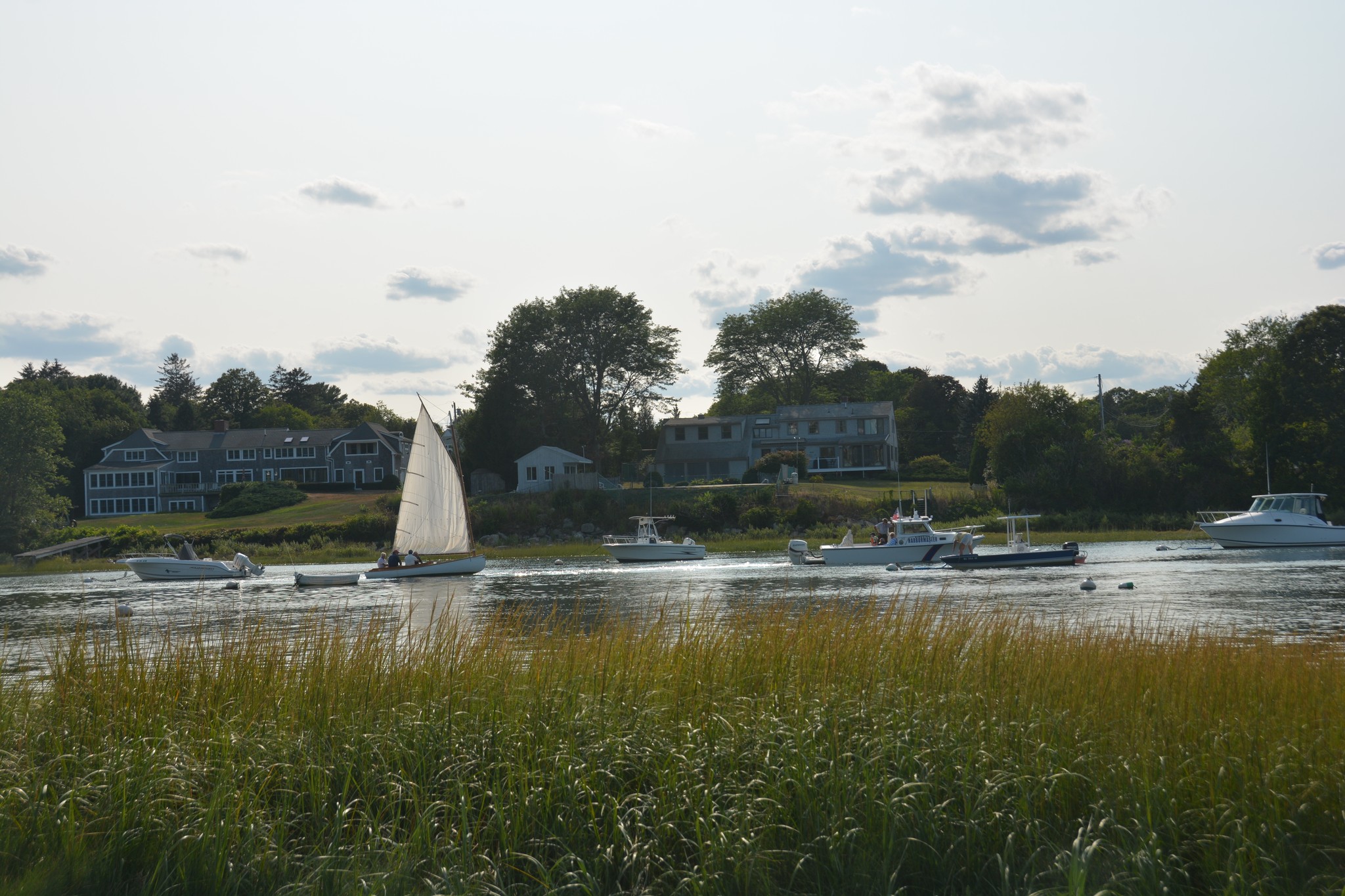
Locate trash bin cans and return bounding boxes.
[791,473,798,484]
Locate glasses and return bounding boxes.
[890,535,894,536]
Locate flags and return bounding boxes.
[892,508,900,520]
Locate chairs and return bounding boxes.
[828,459,836,468]
[598,482,606,489]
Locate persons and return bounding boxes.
[869,532,878,546]
[388,550,402,568]
[377,552,388,568]
[412,552,423,565]
[883,532,897,546]
[873,518,895,546]
[878,538,886,546]
[952,532,973,555]
[404,550,419,566]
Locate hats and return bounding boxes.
[408,550,413,554]
[870,532,875,535]
[394,550,400,553]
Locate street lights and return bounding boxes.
[581,445,586,473]
[794,436,800,468]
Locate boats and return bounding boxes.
[787,470,986,565]
[115,534,266,581]
[283,539,361,586]
[601,471,706,562]
[938,498,1088,570]
[1194,440,1345,549]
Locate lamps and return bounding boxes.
[851,408,854,414]
[827,407,831,413]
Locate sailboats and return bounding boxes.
[364,391,486,580]
